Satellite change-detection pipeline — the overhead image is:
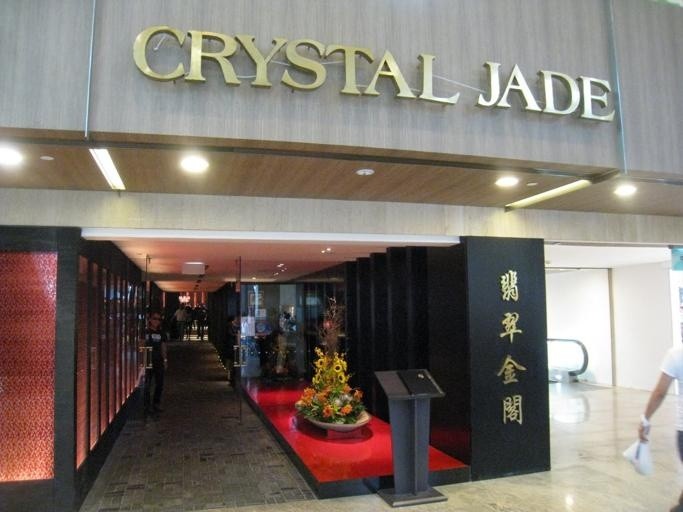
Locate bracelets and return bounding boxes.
[164,359,168,363]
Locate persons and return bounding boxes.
[223,315,241,385]
[256,334,276,369]
[140,313,169,415]
[161,302,209,342]
[637,344,683,512]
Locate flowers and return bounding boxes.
[295,300,365,424]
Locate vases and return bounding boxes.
[295,398,371,432]
